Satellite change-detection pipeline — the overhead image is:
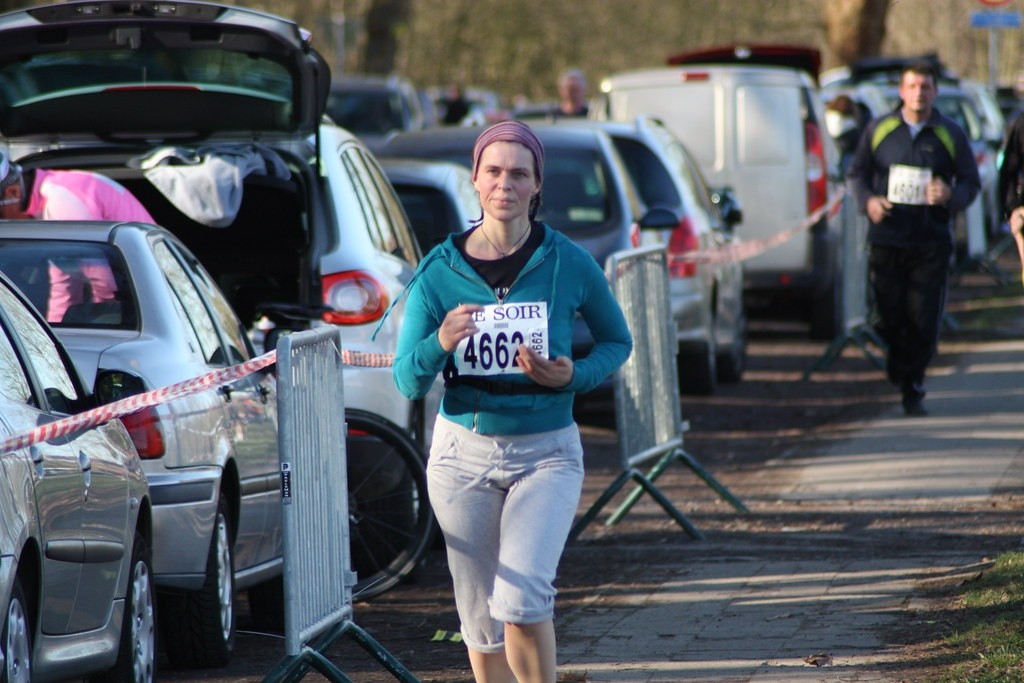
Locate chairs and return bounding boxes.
[64,304,123,329]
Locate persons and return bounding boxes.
[438,69,594,126]
[371,121,633,683]
[829,70,1024,419]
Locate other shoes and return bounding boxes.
[904,388,928,417]
[886,343,905,385]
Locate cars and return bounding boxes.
[318,41,1024,415]
[0,268,160,683]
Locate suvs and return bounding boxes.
[0,217,285,672]
[0,0,439,583]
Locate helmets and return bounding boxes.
[0,148,23,189]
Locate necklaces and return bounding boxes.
[480,222,530,256]
